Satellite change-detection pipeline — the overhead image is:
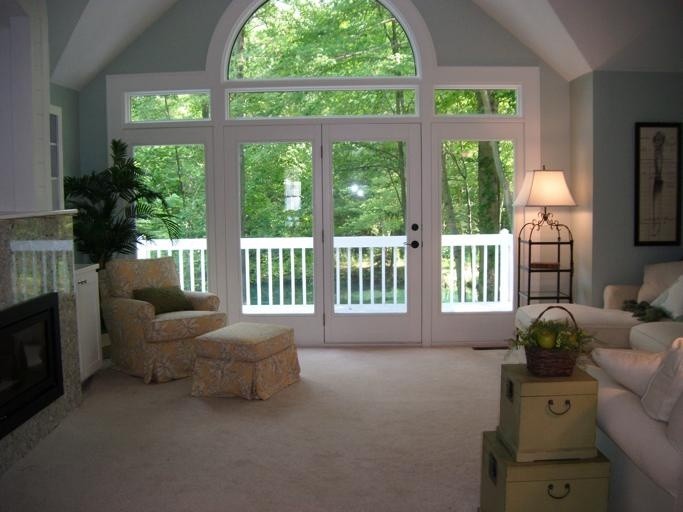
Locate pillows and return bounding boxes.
[635,261,681,305]
[588,346,665,393]
[639,331,681,422]
[130,284,193,315]
[649,267,682,321]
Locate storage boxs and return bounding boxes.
[495,362,602,463]
[477,429,613,512]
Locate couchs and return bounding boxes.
[186,319,305,404]
[97,250,231,387]
[506,261,682,512]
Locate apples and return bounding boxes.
[536,329,556,349]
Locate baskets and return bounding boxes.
[525,306,581,376]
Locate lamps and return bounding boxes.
[514,162,578,240]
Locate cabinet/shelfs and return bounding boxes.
[72,259,104,384]
[514,236,576,307]
[47,104,64,211]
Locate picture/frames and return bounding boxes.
[630,119,682,248]
